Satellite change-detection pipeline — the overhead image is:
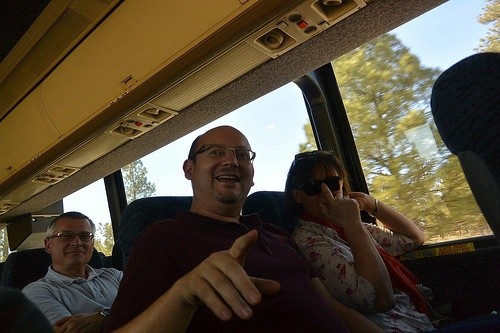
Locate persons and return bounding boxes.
[283,150,500,333]
[103,126,384,333]
[22,212,123,333]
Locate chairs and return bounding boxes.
[430,52,500,239]
[117,196,197,266]
[0,246,105,292]
[242,191,301,234]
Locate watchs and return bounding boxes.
[100,306,111,318]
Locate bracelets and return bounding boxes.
[369,199,379,217]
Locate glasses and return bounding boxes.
[191,143,256,164]
[48,232,96,242]
[298,175,345,197]
[295,149,335,161]
